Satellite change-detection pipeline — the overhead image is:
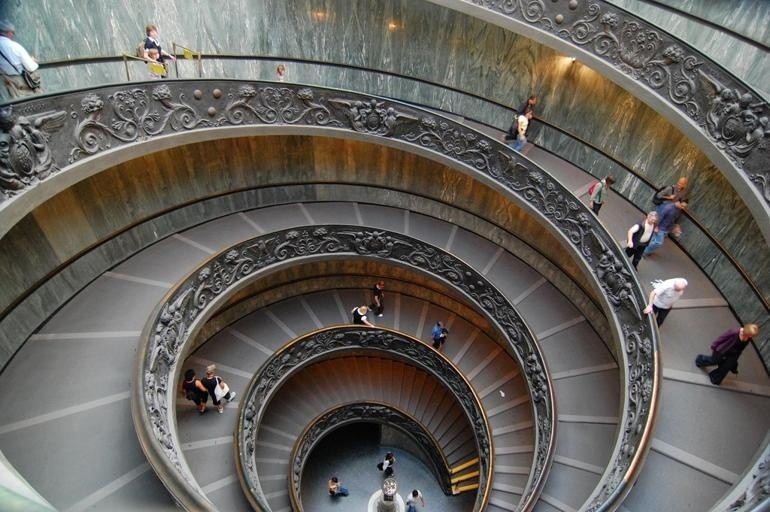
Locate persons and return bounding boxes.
[181,364,236,414]
[382,452,395,478]
[350,280,385,328]
[695,323,760,385]
[431,321,448,353]
[276,64,288,82]
[642,277,687,329]
[586,174,691,272]
[406,489,424,512]
[0,20,43,103]
[328,476,348,498]
[501,94,538,152]
[140,24,176,80]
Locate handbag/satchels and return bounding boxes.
[149,62,166,76]
[214,383,230,402]
[23,71,40,90]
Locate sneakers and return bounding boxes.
[227,392,236,402]
[218,407,223,413]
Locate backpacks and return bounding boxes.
[589,181,604,196]
[652,185,674,205]
[136,39,149,58]
